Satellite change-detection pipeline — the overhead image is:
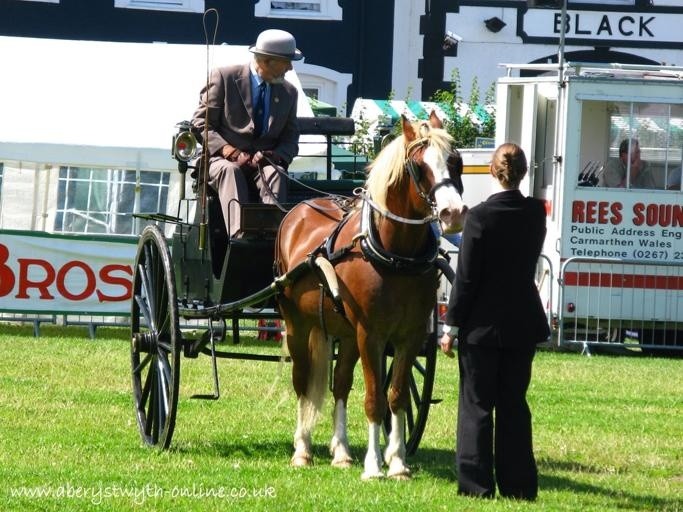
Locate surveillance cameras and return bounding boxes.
[445,31,464,44]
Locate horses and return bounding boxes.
[274,109,468,480]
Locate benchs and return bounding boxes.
[207,115,368,219]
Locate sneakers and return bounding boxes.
[230,231,261,242]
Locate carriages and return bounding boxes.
[122,108,469,482]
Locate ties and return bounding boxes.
[252,81,266,144]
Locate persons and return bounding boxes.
[191,28,303,238]
[440,143,550,498]
[665,166,681,190]
[597,138,656,189]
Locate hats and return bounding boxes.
[248,29,304,61]
